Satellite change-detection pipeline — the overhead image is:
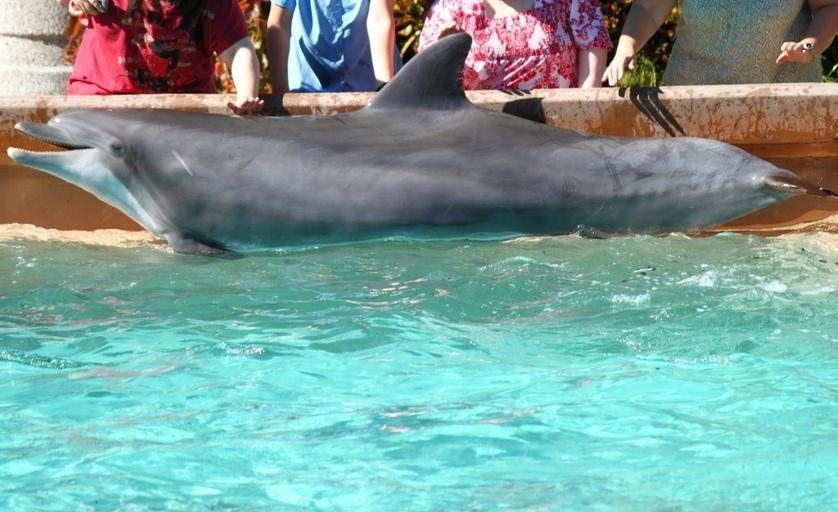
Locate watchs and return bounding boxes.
[801,41,816,57]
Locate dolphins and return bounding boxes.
[6,32,838,255]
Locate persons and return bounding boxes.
[56,0,265,116]
[265,0,405,92]
[416,0,614,91]
[600,1,838,89]
